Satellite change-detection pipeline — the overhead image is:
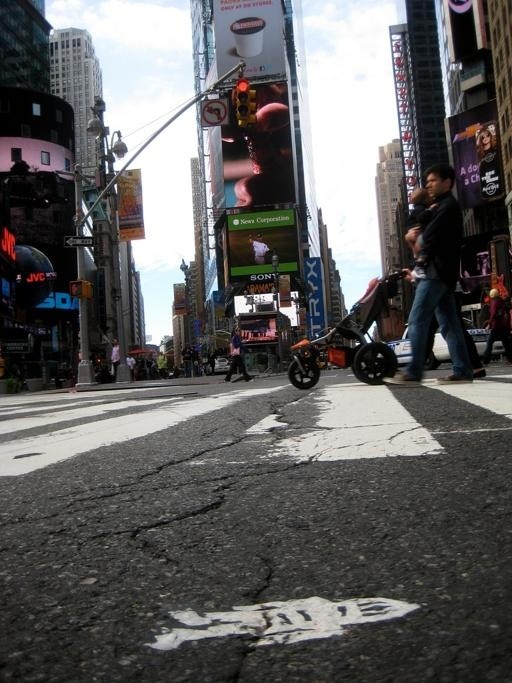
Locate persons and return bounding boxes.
[248,233,270,264]
[127,342,215,380]
[475,128,497,158]
[391,161,474,384]
[424,276,488,380]
[110,336,120,381]
[405,187,437,279]
[224,81,290,208]
[10,363,25,392]
[224,326,253,384]
[484,288,512,364]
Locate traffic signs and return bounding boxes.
[64,234,94,246]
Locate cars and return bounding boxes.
[205,358,230,376]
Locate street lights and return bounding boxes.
[87,94,135,383]
[180,257,192,377]
[270,249,283,373]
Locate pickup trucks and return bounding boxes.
[377,317,504,365]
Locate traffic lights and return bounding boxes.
[248,89,257,122]
[70,281,83,296]
[237,79,251,119]
[84,281,94,298]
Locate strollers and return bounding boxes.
[285,267,408,389]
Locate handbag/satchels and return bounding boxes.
[229,342,240,357]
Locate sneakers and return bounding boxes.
[382,371,422,386]
[410,266,426,280]
[434,374,475,385]
[502,360,511,367]
[471,368,487,379]
[424,358,440,372]
